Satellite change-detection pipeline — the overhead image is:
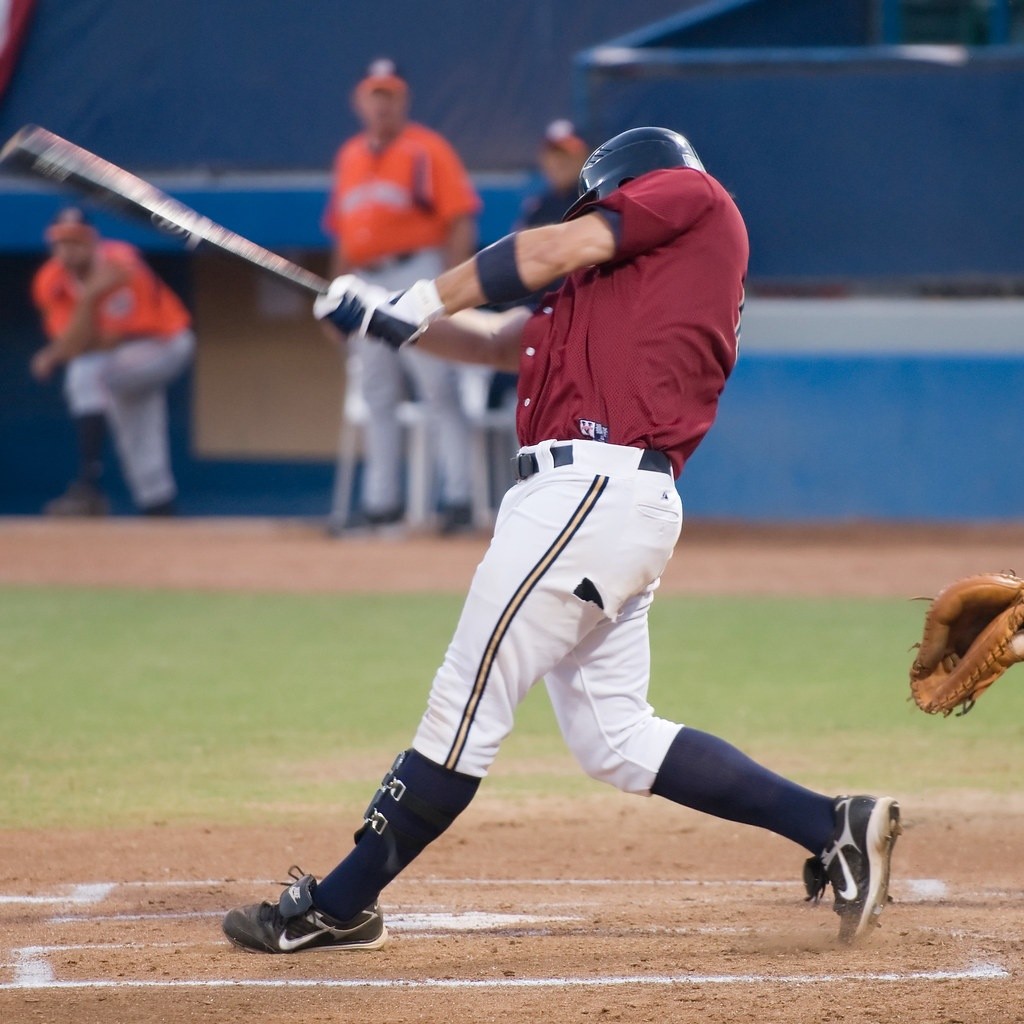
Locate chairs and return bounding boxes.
[327,356,521,537]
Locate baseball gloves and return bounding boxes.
[907,571,1022,720]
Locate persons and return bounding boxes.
[30,207,196,521]
[318,57,482,538]
[488,118,591,315]
[220,129,905,957]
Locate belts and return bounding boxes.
[517,445,671,478]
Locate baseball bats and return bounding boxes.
[0,114,339,312]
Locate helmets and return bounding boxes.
[560,127,705,222]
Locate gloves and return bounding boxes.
[314,273,381,336]
[365,280,444,351]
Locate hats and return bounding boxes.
[539,120,578,154]
[40,207,101,240]
[355,59,404,93]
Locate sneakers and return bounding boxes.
[804,794,902,948]
[223,866,388,954]
